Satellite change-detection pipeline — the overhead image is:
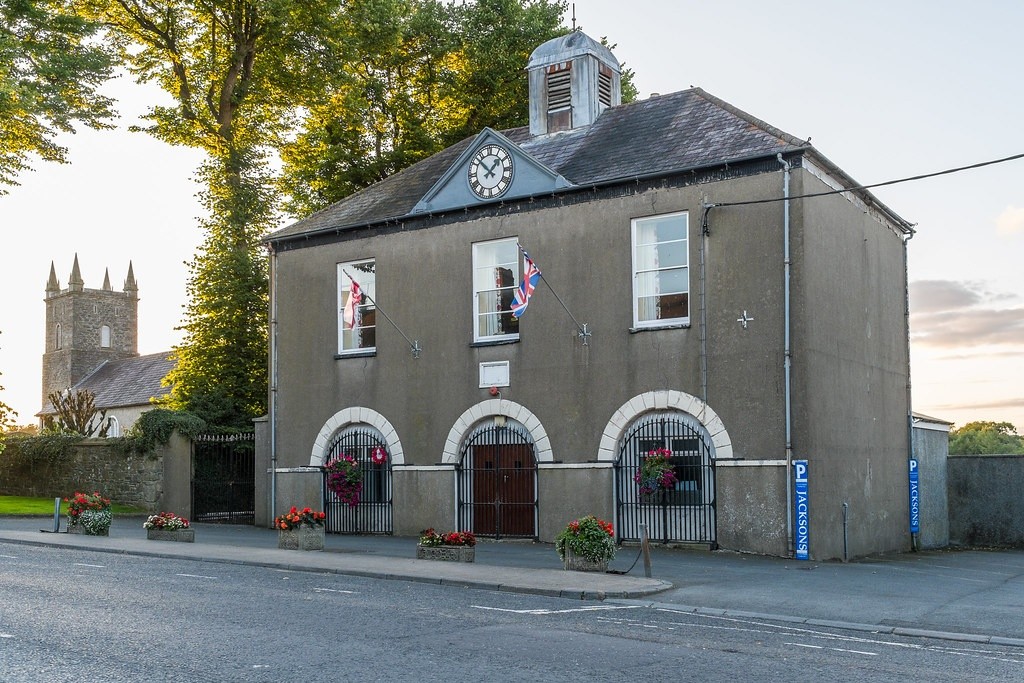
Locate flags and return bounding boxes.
[507,247,542,317]
[344,274,365,326]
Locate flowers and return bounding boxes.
[142,512,191,530]
[633,450,677,495]
[421,531,475,550]
[557,516,614,563]
[64,490,111,519]
[324,453,364,508]
[274,506,326,530]
